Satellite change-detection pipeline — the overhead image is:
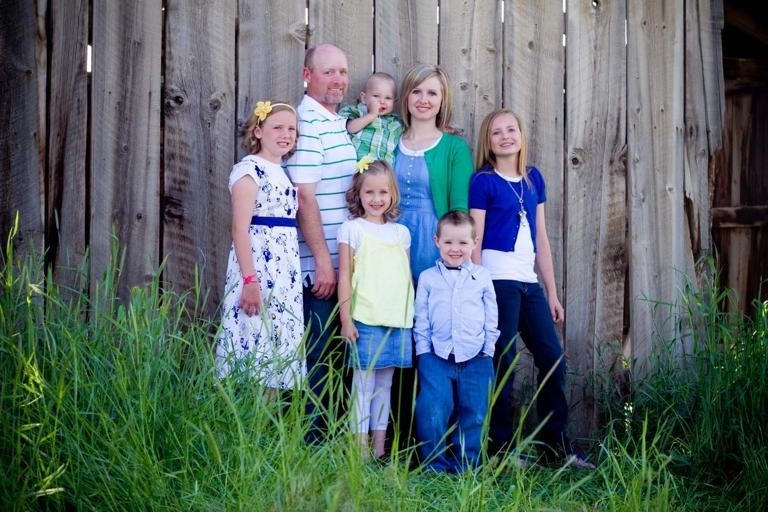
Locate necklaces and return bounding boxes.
[493,163,527,227]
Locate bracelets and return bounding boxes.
[242,272,259,288]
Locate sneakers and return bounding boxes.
[549,451,599,470]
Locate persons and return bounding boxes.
[382,64,475,473]
[336,156,414,464]
[337,72,405,173]
[211,98,310,422]
[279,41,357,448]
[413,208,499,474]
[468,110,599,472]
